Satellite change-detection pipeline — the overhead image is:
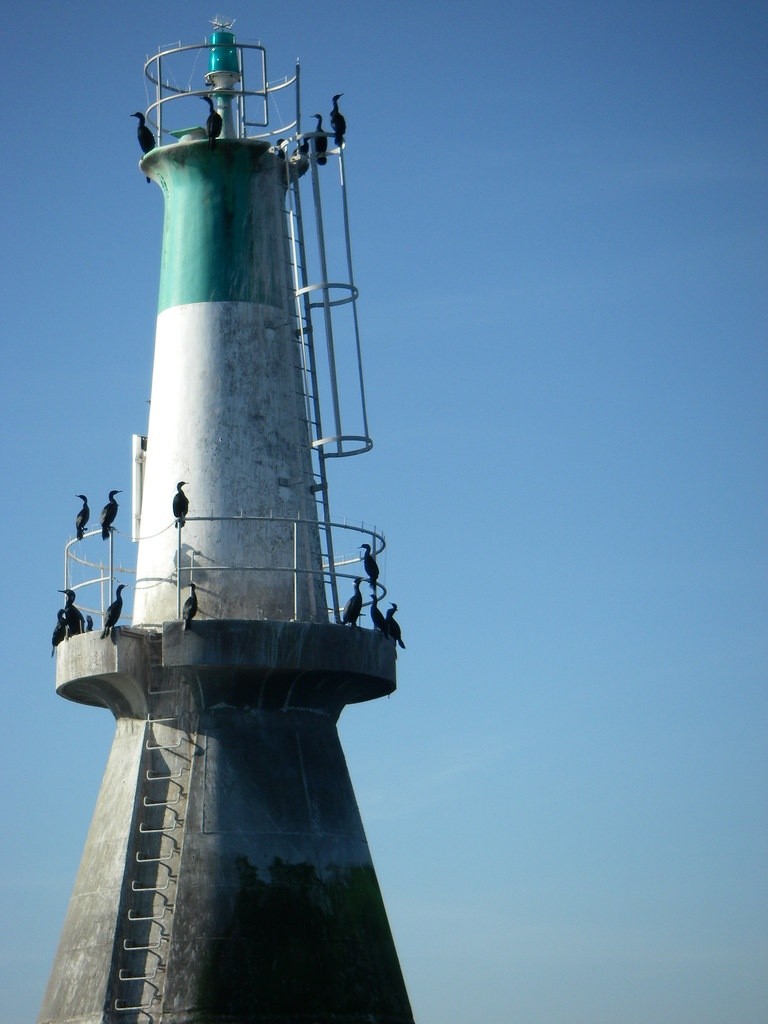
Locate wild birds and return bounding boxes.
[99,584,127,639]
[101,490,124,541]
[369,594,407,650]
[51,589,93,657]
[180,583,198,631]
[308,93,348,166]
[172,481,190,525]
[341,577,364,628]
[129,111,156,185]
[199,94,222,148]
[358,544,380,588]
[74,494,91,541]
